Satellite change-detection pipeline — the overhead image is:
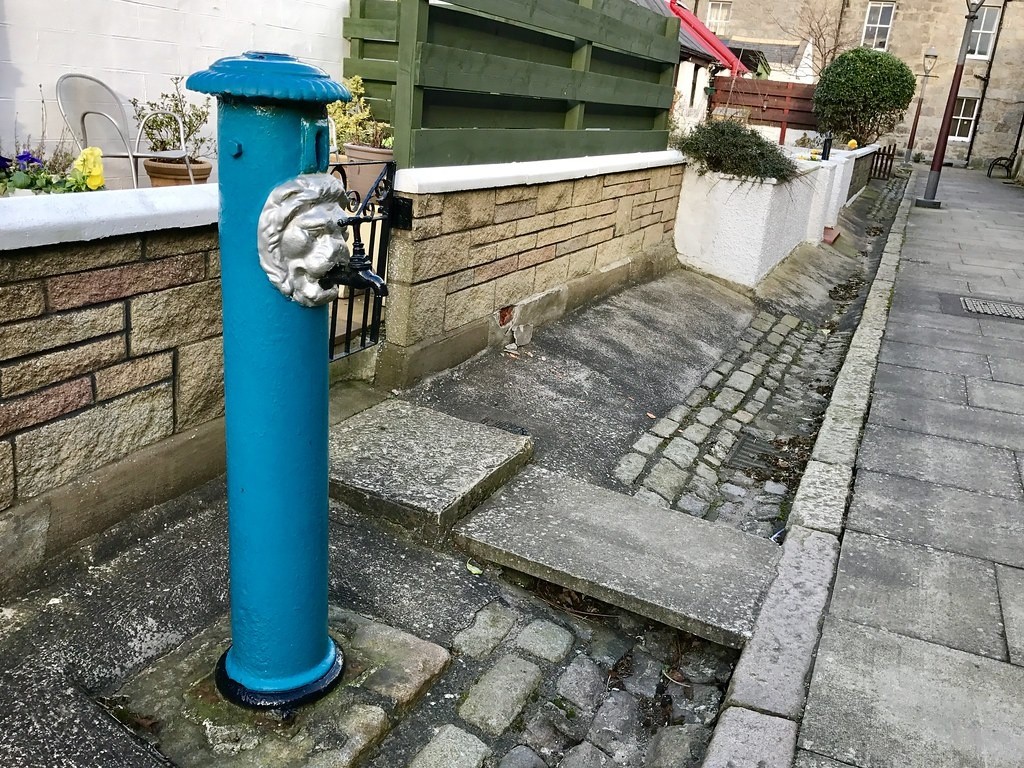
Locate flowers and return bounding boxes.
[0,84,108,197]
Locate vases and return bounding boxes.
[9,188,53,197]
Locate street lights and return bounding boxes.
[915,0,986,210]
[900,47,940,169]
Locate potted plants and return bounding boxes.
[326,74,394,204]
[128,77,218,187]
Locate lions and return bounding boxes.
[257,173,351,311]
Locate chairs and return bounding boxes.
[986,152,1017,178]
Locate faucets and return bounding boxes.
[318,216,390,299]
